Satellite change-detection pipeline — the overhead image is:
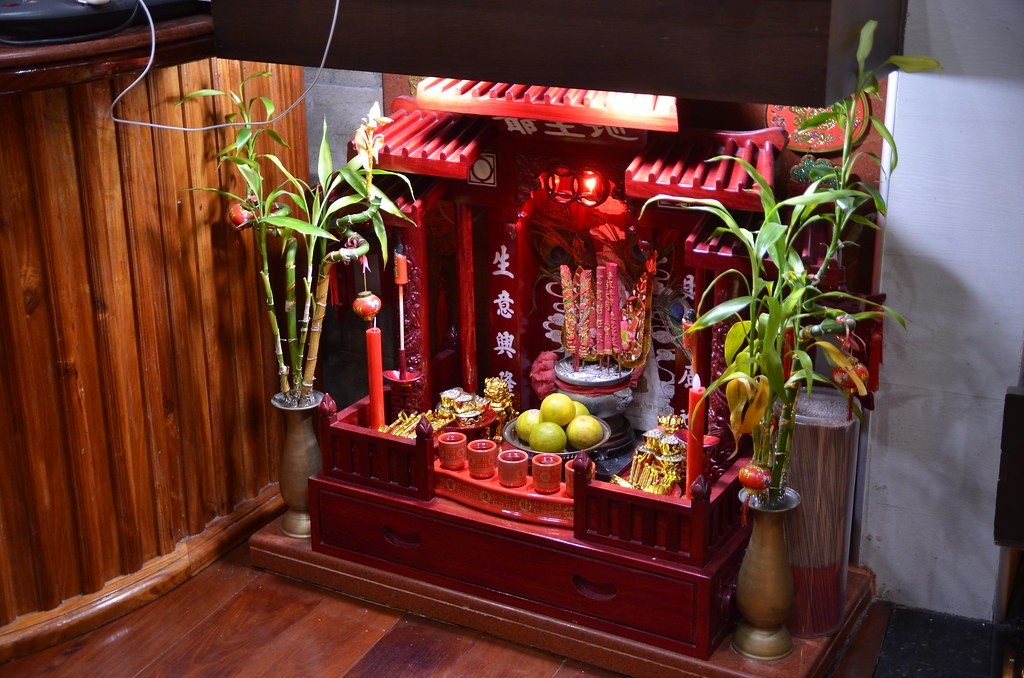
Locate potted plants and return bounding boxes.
[173,69,418,538]
[637,20,945,662]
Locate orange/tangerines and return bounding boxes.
[515,392,603,454]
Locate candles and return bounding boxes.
[686,373,705,500]
[366,317,386,432]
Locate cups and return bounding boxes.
[532,454,562,493]
[564,459,586,497]
[467,439,497,478]
[498,450,528,487]
[438,432,467,469]
